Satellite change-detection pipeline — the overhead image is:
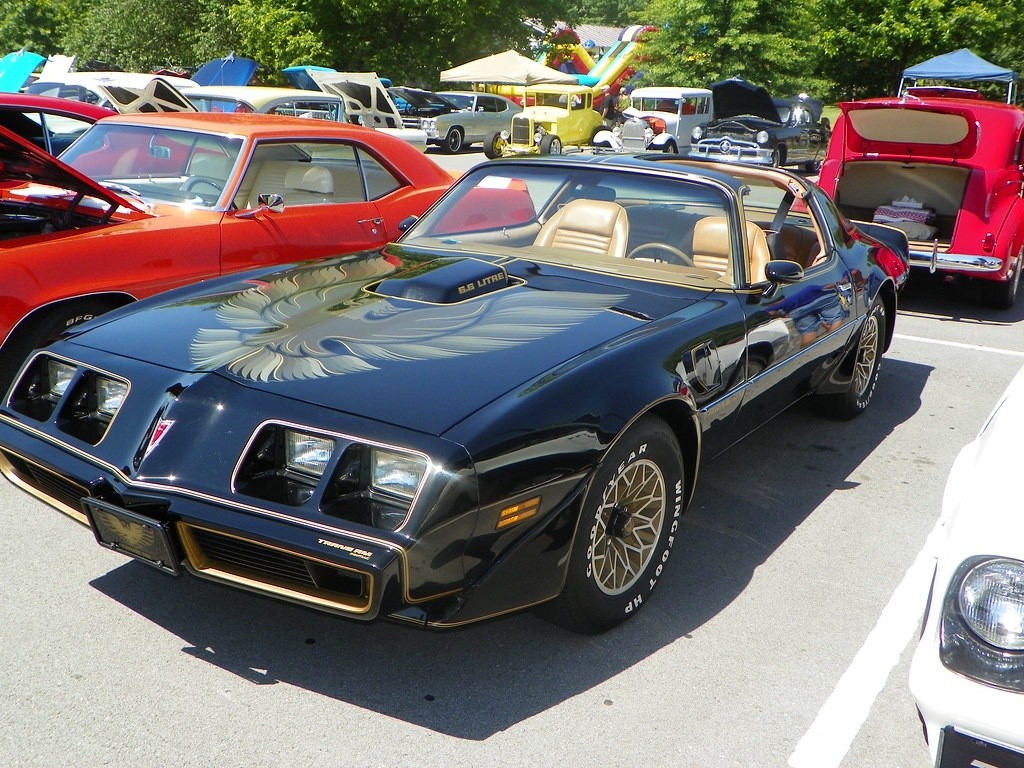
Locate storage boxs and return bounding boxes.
[872,205,936,228]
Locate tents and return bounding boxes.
[897,47,1019,106]
[439,50,580,108]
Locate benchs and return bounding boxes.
[623,206,821,270]
[231,156,397,210]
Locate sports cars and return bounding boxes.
[0,153,911,635]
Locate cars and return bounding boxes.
[0,112,536,404]
[21,72,206,134]
[908,365,1024,768]
[0,92,223,180]
[688,76,833,174]
[789,86,1024,311]
[591,86,714,154]
[387,86,523,155]
[96,77,427,162]
[482,84,603,159]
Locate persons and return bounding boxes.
[614,87,630,124]
[558,93,582,108]
[599,84,614,128]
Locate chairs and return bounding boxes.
[674,105,694,115]
[281,164,335,207]
[660,101,674,114]
[533,198,629,259]
[670,216,771,285]
[179,153,237,205]
